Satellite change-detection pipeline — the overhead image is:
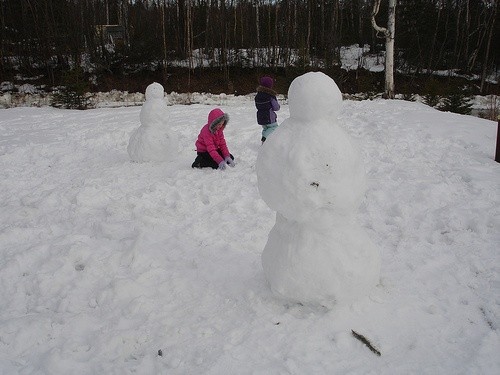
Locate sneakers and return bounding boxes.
[192,155,202,168]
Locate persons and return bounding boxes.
[254,77,280,144]
[192,108,234,170]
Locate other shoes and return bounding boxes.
[262,137,266,142]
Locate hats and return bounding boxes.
[261,77,273,88]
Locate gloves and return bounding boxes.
[225,157,235,167]
[219,160,230,170]
